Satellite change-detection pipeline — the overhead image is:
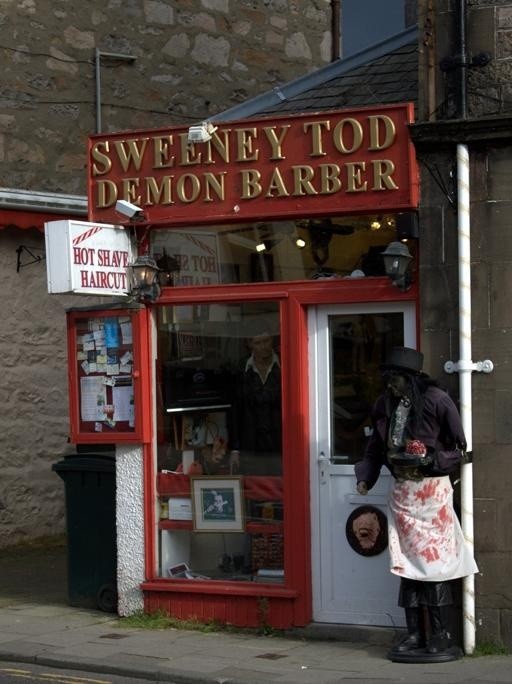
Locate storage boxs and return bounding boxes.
[169,496,193,521]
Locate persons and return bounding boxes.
[224,318,281,578]
[354,346,479,653]
[204,492,234,519]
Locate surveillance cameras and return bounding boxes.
[115,199,145,223]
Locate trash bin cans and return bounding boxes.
[51,451,119,612]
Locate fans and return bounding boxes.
[217,220,357,238]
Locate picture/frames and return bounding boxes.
[190,476,246,534]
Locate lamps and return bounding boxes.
[187,120,218,144]
[123,255,163,306]
[382,241,416,292]
[254,227,309,253]
[370,213,397,232]
